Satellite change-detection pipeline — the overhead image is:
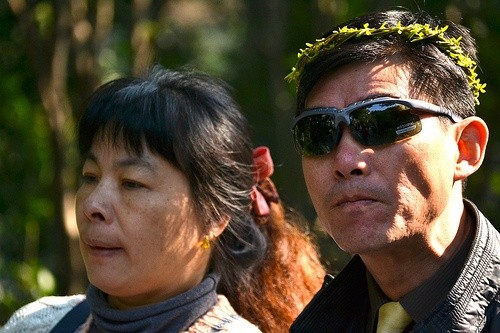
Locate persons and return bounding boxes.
[289,9,500,333]
[0,67,325,333]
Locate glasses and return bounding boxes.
[295,97,465,157]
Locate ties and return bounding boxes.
[376,300,414,333]
